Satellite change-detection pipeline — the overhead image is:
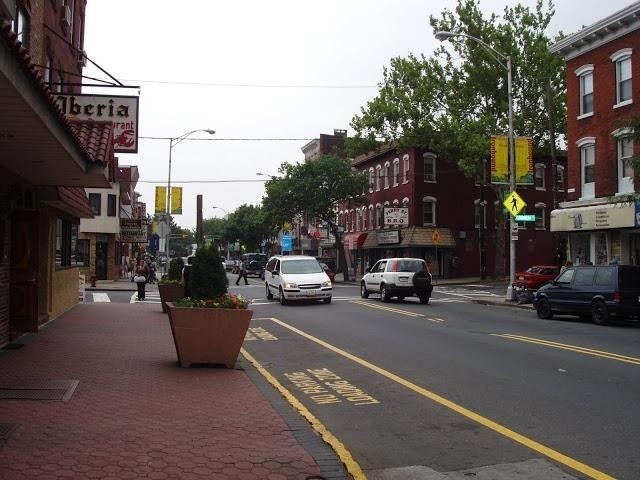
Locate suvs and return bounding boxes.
[242,253,267,275]
[361,256,433,304]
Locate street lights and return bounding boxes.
[165,129,216,279]
[434,32,518,304]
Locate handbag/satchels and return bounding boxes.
[134,275,146,283]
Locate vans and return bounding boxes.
[532,265,640,329]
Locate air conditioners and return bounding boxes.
[77,50,88,67]
[59,3,74,28]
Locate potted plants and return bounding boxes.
[157,237,254,369]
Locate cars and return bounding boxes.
[264,253,333,306]
[516,265,560,287]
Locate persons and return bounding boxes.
[134,260,150,300]
[236,258,250,287]
[121,253,137,279]
